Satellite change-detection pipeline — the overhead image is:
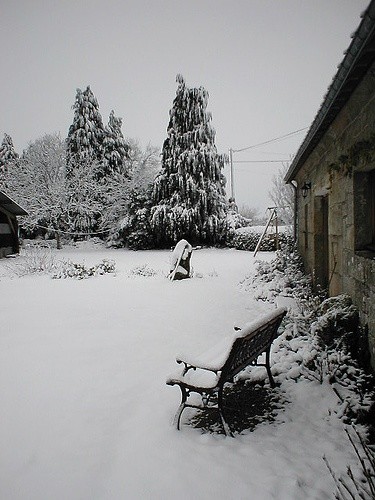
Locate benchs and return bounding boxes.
[165,306,288,441]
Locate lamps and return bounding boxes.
[299,181,311,200]
